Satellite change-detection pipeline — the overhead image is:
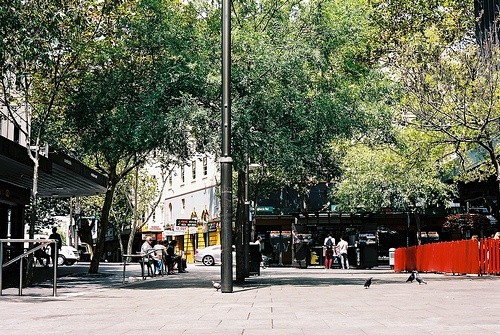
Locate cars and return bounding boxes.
[24,234,80,266]
[193,244,268,266]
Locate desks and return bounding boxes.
[123,254,147,281]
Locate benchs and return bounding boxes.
[136,251,183,277]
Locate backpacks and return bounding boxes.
[326,237,333,252]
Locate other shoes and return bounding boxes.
[167,271,173,275]
[171,270,175,275]
[178,269,185,273]
[183,268,189,273]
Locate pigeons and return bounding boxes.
[405,271,416,284]
[416,276,428,286]
[363,277,373,289]
[211,280,221,291]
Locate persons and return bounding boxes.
[337,235,350,270]
[33,238,50,267]
[254,236,263,262]
[49,227,62,268]
[153,240,189,276]
[470,231,500,261]
[324,231,336,269]
[141,236,163,277]
